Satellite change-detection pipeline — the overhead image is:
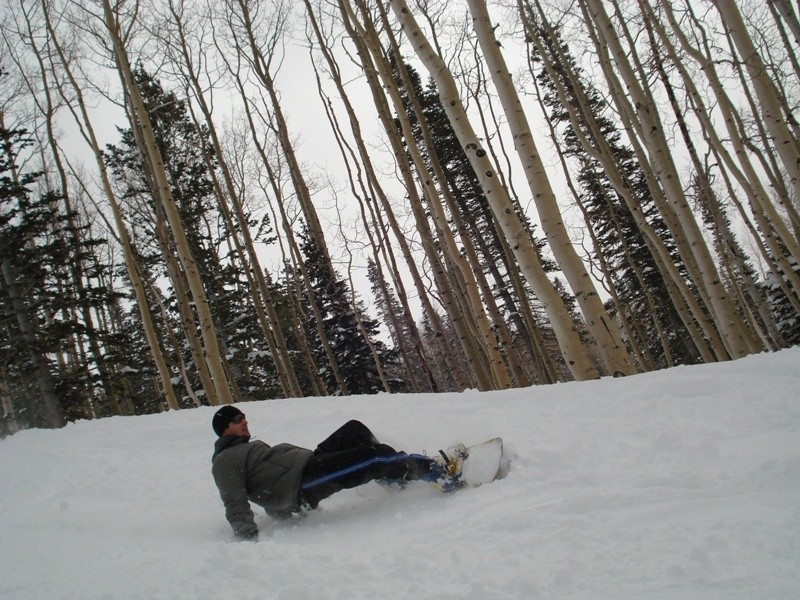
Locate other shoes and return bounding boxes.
[433,442,465,477]
[430,474,460,494]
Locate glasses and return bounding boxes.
[231,413,246,424]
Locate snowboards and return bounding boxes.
[429,436,504,489]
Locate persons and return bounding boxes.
[211,406,466,543]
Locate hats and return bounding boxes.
[212,405,244,437]
